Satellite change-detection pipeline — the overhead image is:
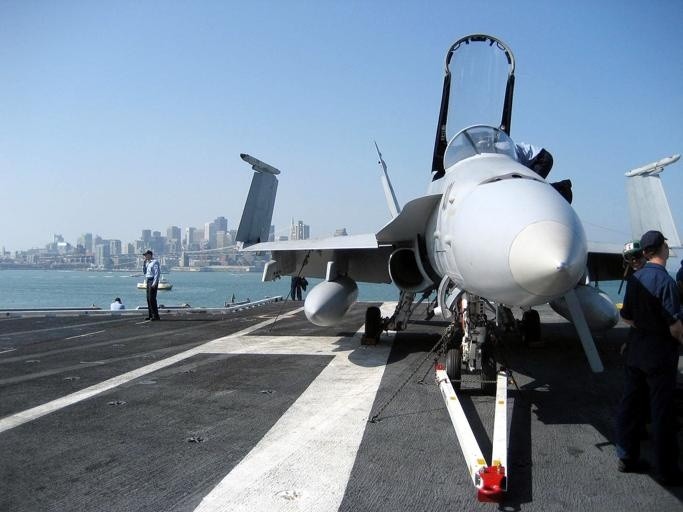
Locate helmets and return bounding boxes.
[621,239,648,271]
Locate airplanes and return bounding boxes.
[236,34,683,386]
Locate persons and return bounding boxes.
[675,260,683,284]
[111,298,124,310]
[616,231,682,474]
[623,240,644,268]
[142,250,160,321]
[291,276,302,300]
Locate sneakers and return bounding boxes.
[655,465,683,486]
[618,455,650,473]
[150,317,161,321]
[145,317,153,320]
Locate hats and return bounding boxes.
[640,230,668,255]
[115,298,121,302]
[143,250,153,256]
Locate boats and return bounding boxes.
[130,272,145,277]
[137,277,173,291]
